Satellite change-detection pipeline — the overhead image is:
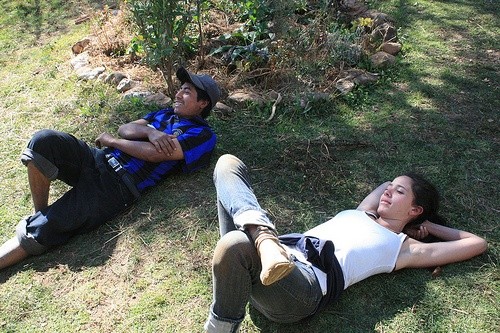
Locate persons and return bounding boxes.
[204,154,488,333]
[0,67,221,271]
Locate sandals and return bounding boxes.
[252,225,296,285]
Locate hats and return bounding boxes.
[175,66,220,106]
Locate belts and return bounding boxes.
[103,150,140,198]
